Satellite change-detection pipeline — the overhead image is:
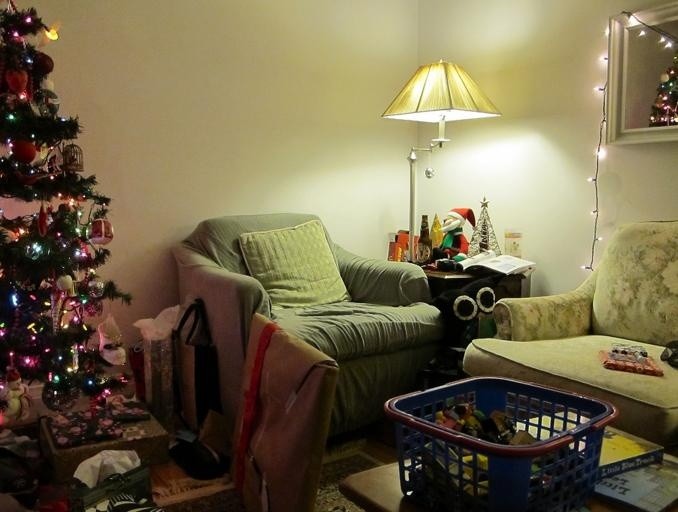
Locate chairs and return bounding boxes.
[462,217,678,456]
[173,212,446,441]
[153,309,341,509]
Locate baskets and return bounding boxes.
[388,377,619,505]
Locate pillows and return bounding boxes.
[237,218,354,314]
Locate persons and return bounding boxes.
[0,364,29,418]
[431,208,477,263]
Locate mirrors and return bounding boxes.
[605,2,678,146]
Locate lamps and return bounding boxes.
[380,59,503,261]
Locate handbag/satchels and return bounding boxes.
[68,459,155,510]
[135,326,171,410]
[173,300,216,432]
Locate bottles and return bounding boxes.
[504,233,523,259]
[416,214,433,263]
